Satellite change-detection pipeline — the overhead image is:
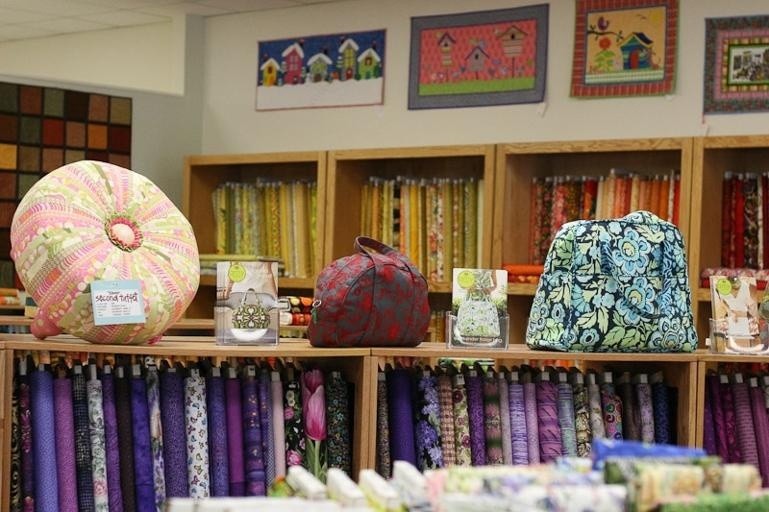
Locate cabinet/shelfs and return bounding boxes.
[0,312,768,511]
[180,137,769,337]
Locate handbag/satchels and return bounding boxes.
[232,305,269,329]
[308,236,430,348]
[526,210,698,352]
[457,301,500,337]
